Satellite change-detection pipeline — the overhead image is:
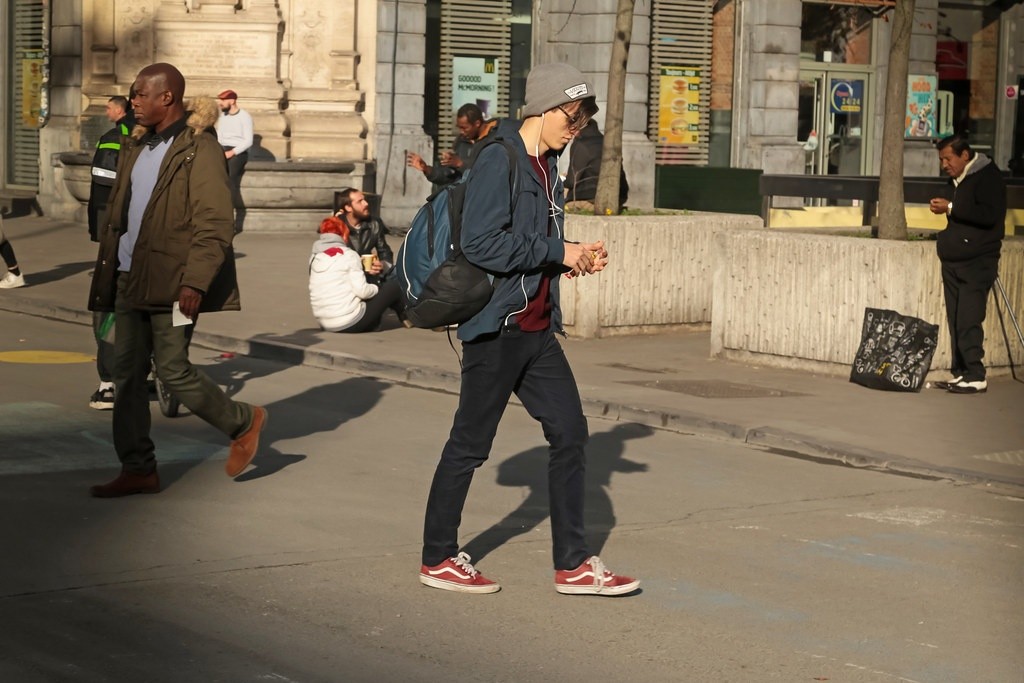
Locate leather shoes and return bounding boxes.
[90,468,160,497]
[226,404,267,476]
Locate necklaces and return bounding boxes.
[348,215,360,234]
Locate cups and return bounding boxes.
[361,254,374,271]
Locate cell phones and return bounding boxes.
[502,323,521,337]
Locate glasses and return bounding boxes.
[554,104,582,129]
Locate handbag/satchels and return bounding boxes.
[849,307,939,393]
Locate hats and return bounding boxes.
[522,64,596,120]
[218,90,237,100]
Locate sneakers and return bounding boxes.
[420,552,501,593]
[934,375,964,389]
[948,379,988,393]
[89,388,114,409]
[554,555,641,596]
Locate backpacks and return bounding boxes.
[396,138,520,327]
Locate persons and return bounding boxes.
[419,62,641,596]
[106,96,130,129]
[88,83,139,411]
[215,90,254,236]
[556,118,629,207]
[333,187,447,332]
[0,213,30,289]
[930,133,1009,394]
[87,63,268,499]
[308,216,416,332]
[405,104,505,185]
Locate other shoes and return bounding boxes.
[0,271,26,288]
[404,318,413,330]
